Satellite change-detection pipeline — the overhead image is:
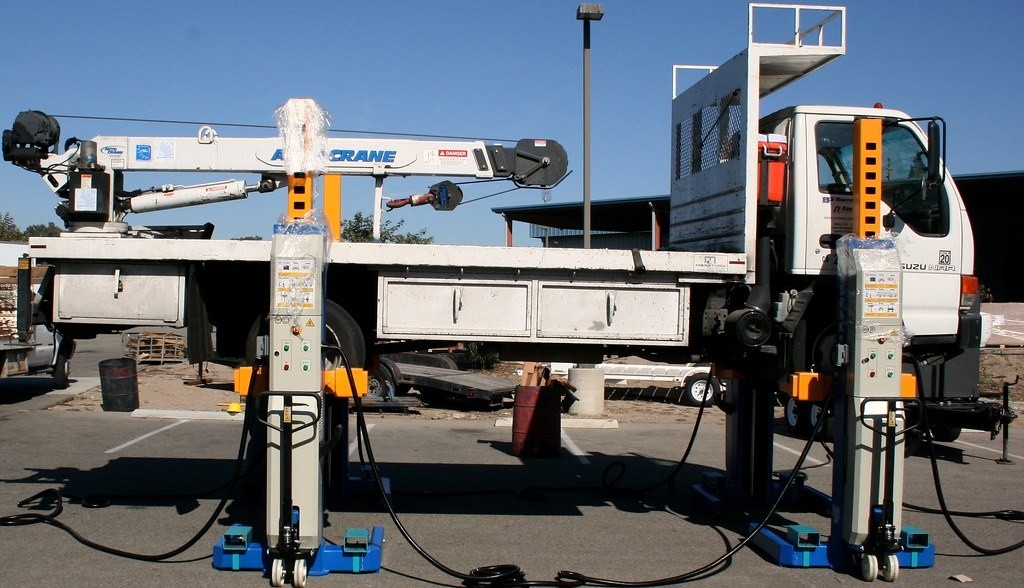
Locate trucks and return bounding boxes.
[0,240,75,389]
[542,360,727,408]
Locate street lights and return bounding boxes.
[574,1,608,249]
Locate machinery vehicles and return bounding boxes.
[0,3,982,371]
